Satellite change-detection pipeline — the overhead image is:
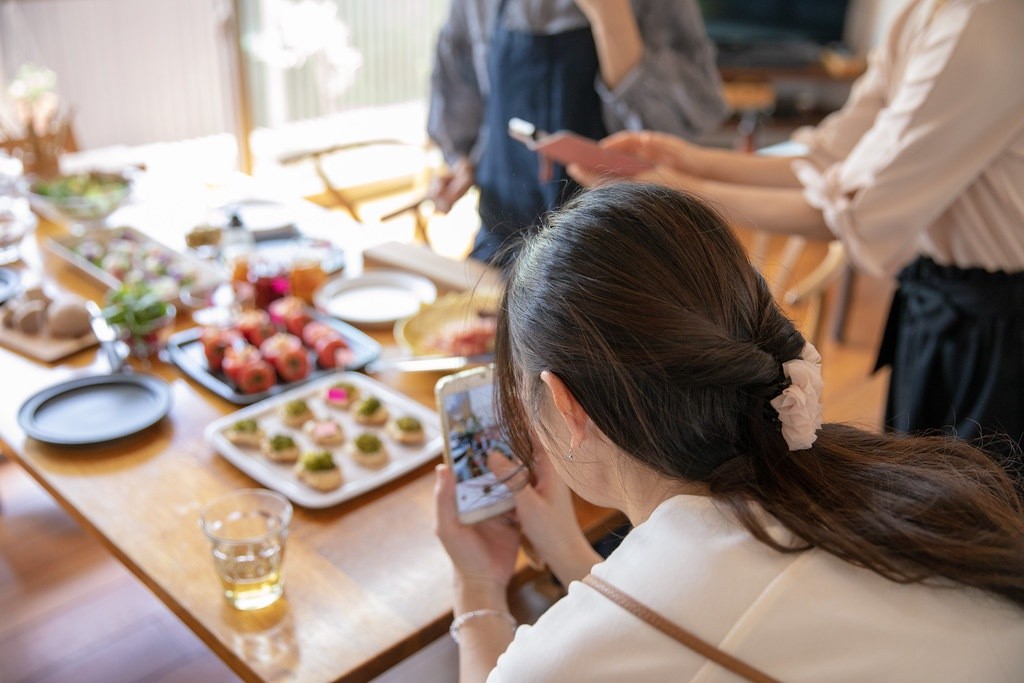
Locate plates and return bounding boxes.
[205,370,443,507]
[312,270,439,325]
[42,226,222,312]
[19,373,169,444]
[21,167,140,218]
[167,302,382,404]
[395,305,477,355]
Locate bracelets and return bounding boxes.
[448,607,519,646]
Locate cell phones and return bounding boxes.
[508,117,648,180]
[434,362,519,525]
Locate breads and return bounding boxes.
[0,284,97,342]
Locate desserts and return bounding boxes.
[203,303,424,495]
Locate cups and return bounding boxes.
[201,488,292,611]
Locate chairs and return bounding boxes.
[274,134,440,248]
[722,144,851,356]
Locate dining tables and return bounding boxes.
[0,175,632,683]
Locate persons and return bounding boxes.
[433,180,1024,683]
[566,1,1023,487]
[423,2,735,268]
